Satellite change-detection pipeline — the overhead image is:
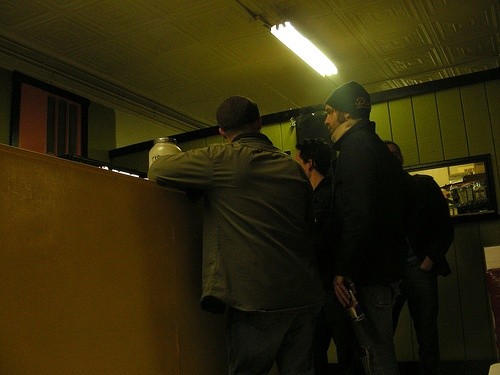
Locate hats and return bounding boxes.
[325,81,371,116]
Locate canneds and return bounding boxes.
[347,289,365,322]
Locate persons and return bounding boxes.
[147,96,314,375]
[384,141,455,375]
[324,81,404,375]
[294,138,332,189]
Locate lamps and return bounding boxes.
[270,16,339,77]
